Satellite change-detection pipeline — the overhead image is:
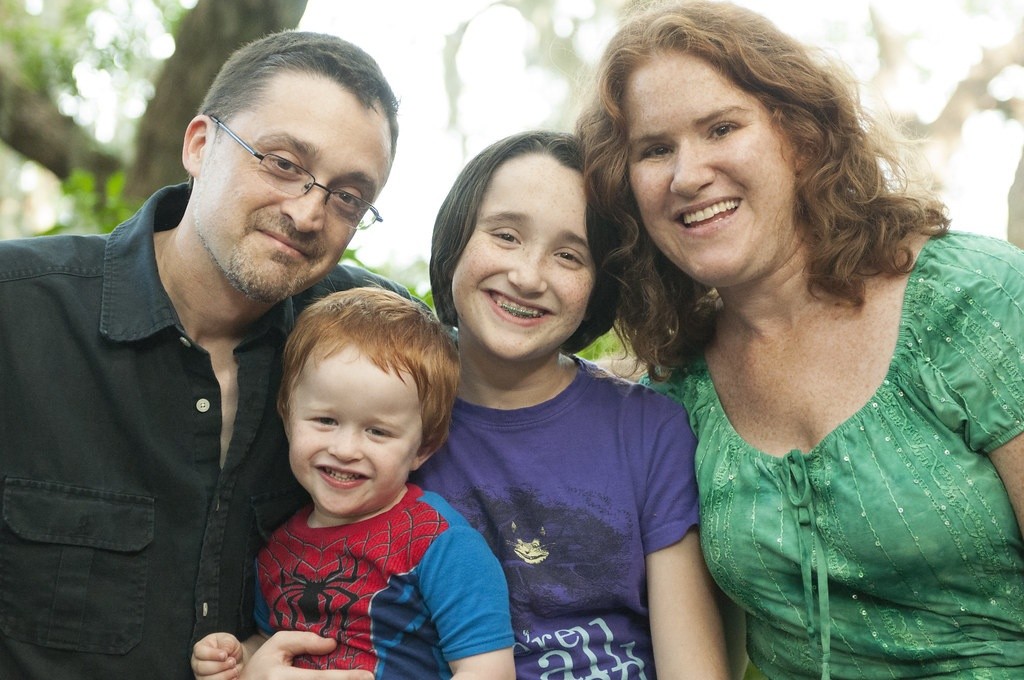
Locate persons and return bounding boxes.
[0,31,435,679]
[407,130,727,680]
[189,287,516,679]
[585,2,1024,680]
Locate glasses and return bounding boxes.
[207,115,384,230]
